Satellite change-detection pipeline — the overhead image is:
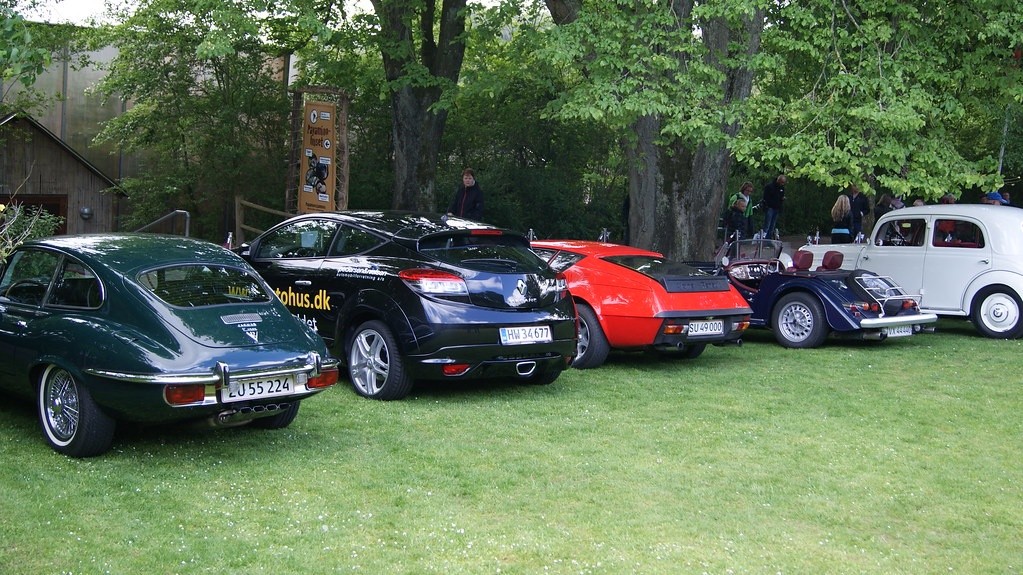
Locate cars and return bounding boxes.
[181,209,578,401]
[681,238,937,350]
[798,204,1023,339]
[0,232,341,457]
[529,239,754,370]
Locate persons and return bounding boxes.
[981,192,1016,208]
[831,195,857,244]
[725,199,748,258]
[872,165,955,246]
[446,168,484,247]
[764,175,786,248]
[847,181,868,244]
[727,181,753,245]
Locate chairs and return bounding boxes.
[786,250,813,274]
[816,250,843,273]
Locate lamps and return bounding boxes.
[79,207,94,219]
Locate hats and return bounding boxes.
[986,192,1007,204]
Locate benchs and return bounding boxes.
[155,278,224,306]
